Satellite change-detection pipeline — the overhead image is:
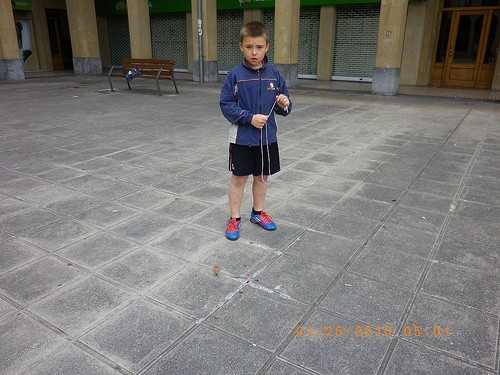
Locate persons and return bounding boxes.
[219,21,292,240]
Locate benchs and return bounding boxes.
[108,59,178,96]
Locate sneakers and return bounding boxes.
[250,209,277,231]
[226,219,242,241]
